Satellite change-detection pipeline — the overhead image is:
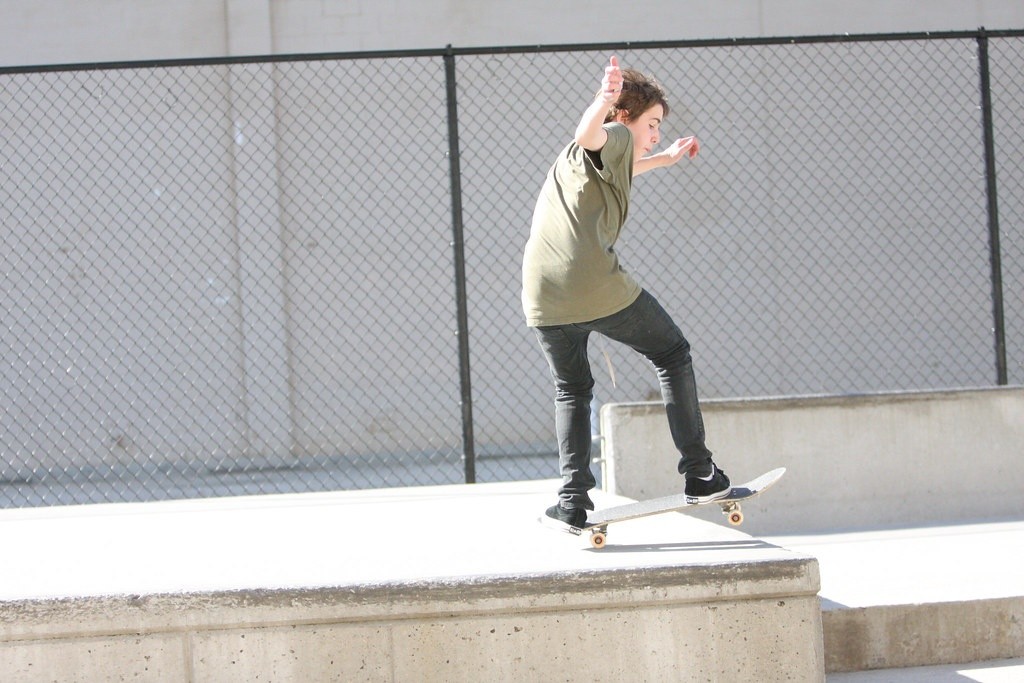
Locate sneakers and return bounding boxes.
[684,462,732,505]
[543,500,587,536]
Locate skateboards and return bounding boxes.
[546,466,788,550]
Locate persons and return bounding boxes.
[521,56,731,536]
